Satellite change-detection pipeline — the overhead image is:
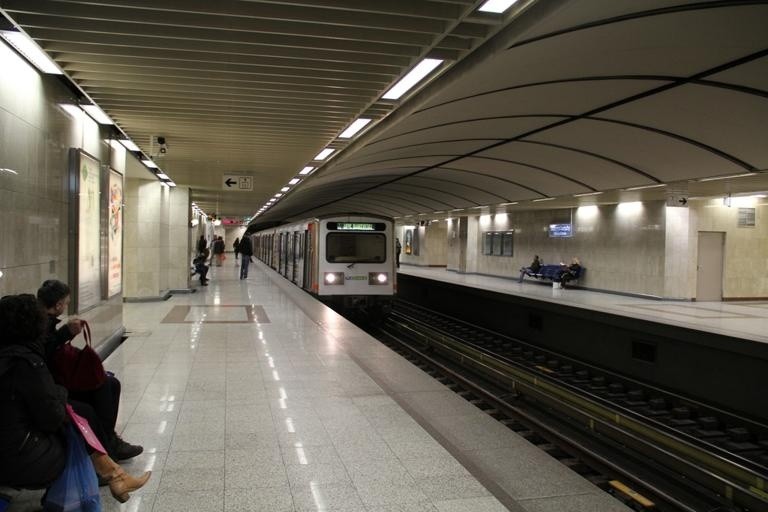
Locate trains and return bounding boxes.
[249,211,399,315]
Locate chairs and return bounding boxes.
[520,260,584,290]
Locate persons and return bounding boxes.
[36,281,144,465]
[518,255,541,283]
[0,294,150,502]
[561,255,580,289]
[192,232,253,285]
[395,237,401,268]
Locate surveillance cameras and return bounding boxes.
[160,143,167,153]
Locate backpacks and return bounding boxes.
[63,320,108,399]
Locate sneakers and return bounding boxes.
[105,438,145,461]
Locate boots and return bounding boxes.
[89,449,152,504]
